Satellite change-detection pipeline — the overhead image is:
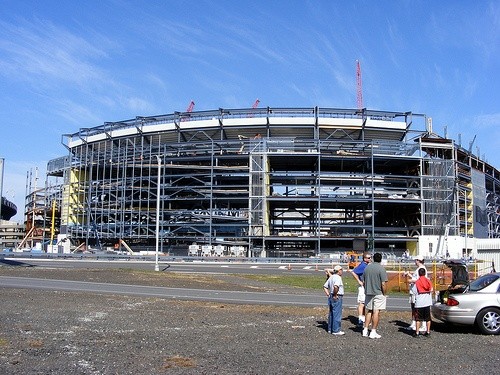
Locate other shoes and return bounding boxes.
[406,325,415,330]
[419,327,427,331]
[414,332,419,338]
[424,332,431,338]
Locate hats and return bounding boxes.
[334,264,342,273]
[414,255,423,260]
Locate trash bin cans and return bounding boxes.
[3,248,12,252]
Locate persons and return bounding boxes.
[351,254,354,262]
[360,253,388,339]
[414,268,433,338]
[403,255,427,332]
[350,252,372,329]
[323,265,345,335]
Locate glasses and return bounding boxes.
[367,257,371,259]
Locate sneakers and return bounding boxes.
[362,329,369,337]
[328,331,345,335]
[369,331,382,339]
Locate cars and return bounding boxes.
[399,254,474,264]
[430,259,500,335]
